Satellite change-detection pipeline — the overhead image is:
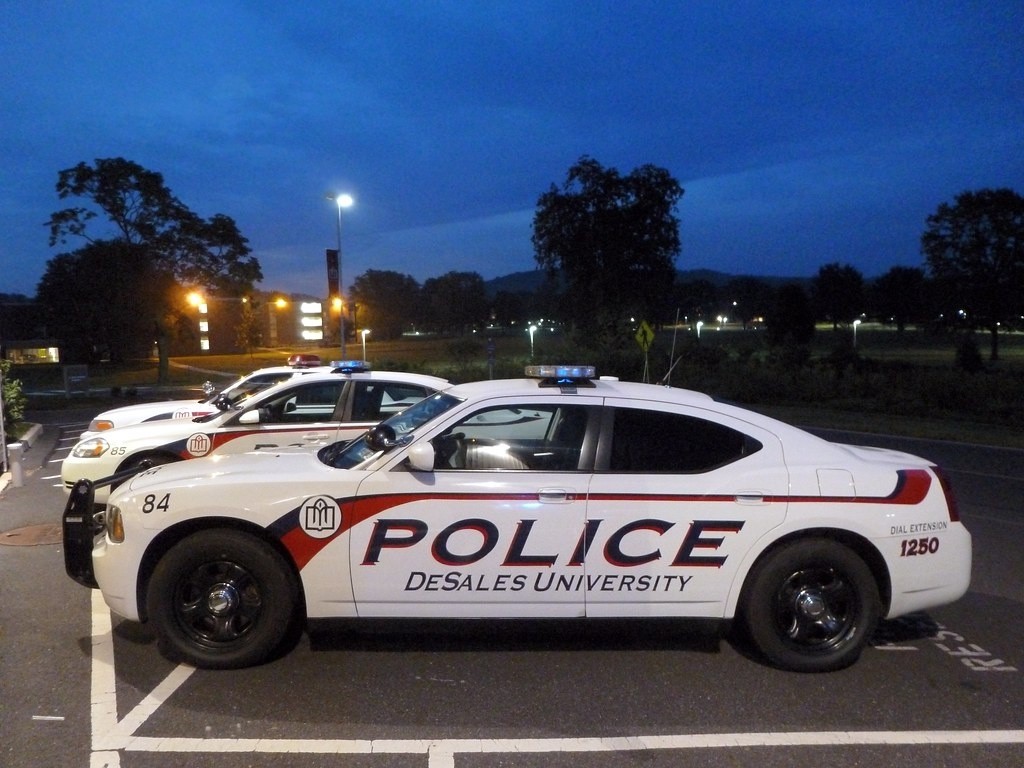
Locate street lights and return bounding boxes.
[336,194,353,361]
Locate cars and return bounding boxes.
[91,362,973,670]
[60,350,554,591]
